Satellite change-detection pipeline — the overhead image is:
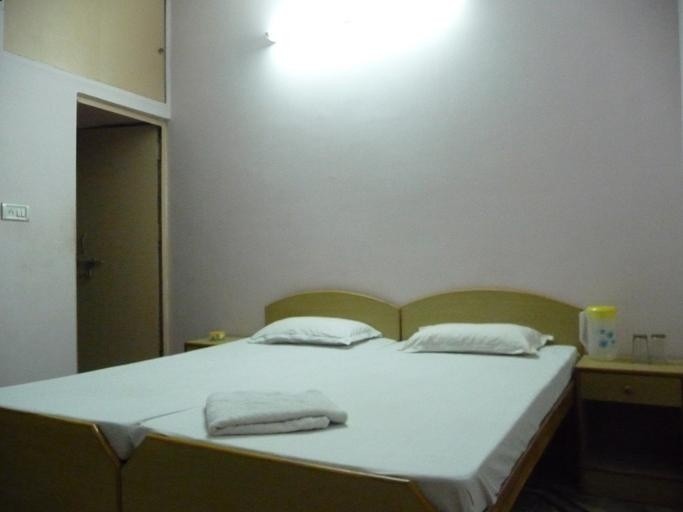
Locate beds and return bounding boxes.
[1,287,400,511]
[115,287,595,512]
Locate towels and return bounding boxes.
[203,389,349,439]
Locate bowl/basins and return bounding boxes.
[208,331,225,340]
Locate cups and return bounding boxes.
[628,330,667,362]
[576,303,620,360]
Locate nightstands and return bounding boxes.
[183,335,244,353]
[574,353,682,484]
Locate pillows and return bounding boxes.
[247,316,384,347]
[397,324,555,358]
